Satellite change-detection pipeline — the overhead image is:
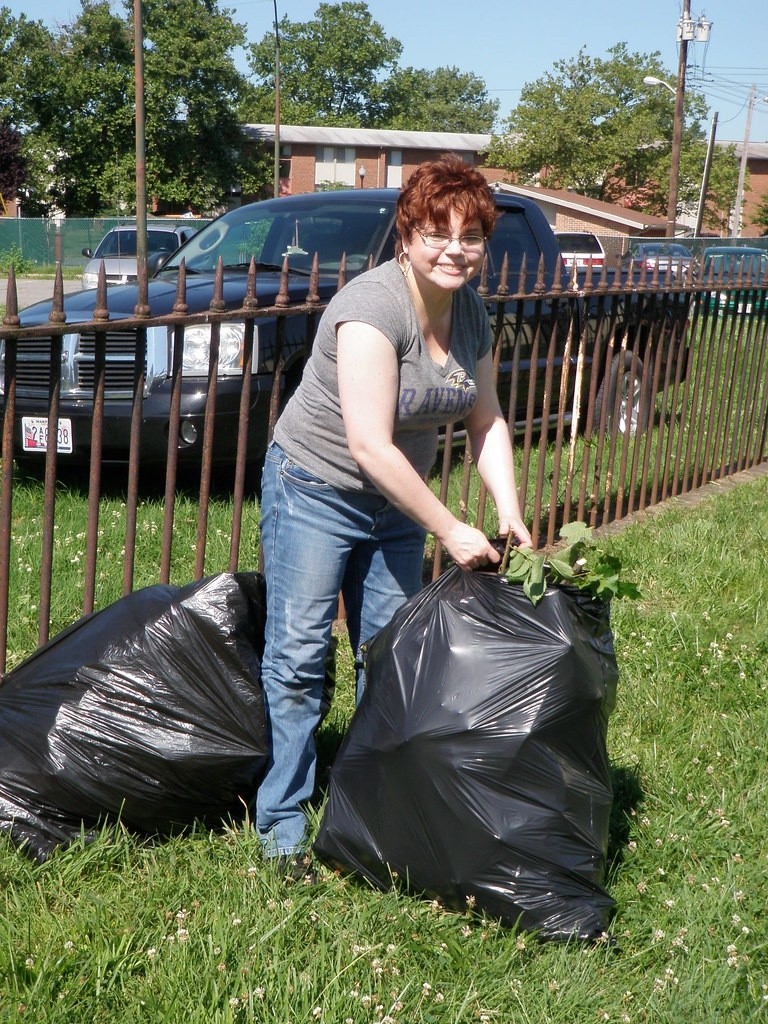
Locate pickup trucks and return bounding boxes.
[0,183,705,497]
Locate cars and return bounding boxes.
[620,242,695,275]
[78,224,201,292]
[693,248,768,320]
[552,232,604,273]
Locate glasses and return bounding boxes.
[412,223,488,250]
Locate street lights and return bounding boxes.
[359,165,367,188]
[643,0,691,240]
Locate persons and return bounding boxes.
[252,152,535,886]
